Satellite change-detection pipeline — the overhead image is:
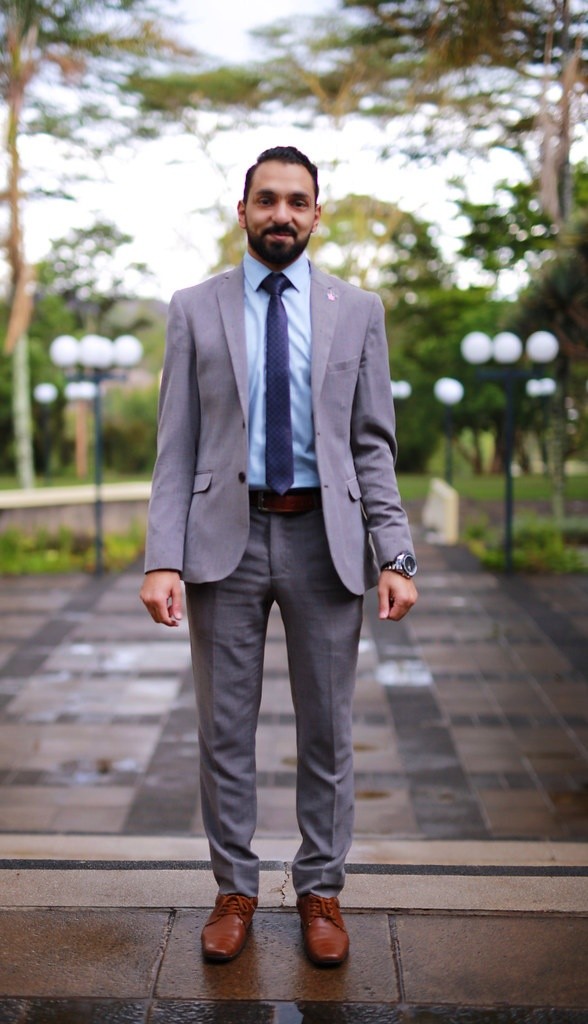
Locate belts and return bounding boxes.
[248,490,323,513]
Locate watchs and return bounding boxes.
[381,550,418,580]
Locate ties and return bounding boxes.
[265,270,295,495]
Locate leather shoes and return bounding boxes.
[296,895,350,964]
[201,893,258,961]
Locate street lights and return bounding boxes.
[430,327,561,578]
[30,333,143,577]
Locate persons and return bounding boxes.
[138,142,423,971]
